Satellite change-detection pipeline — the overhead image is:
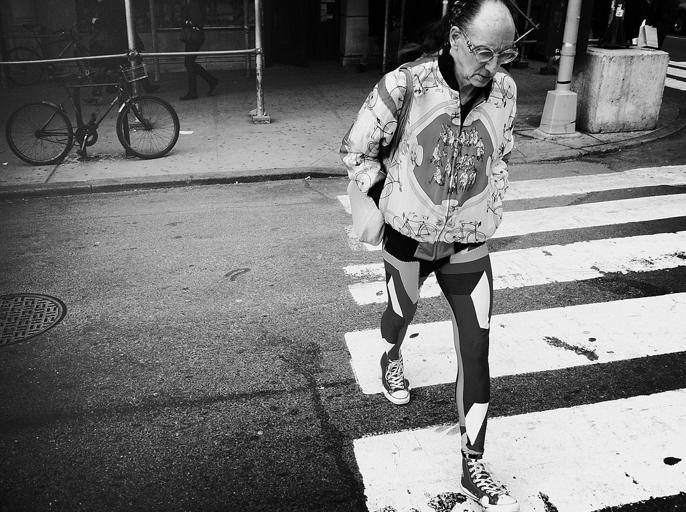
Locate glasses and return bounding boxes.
[461,30,519,65]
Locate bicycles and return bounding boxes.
[4,22,92,86]
[4,46,180,165]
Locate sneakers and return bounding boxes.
[461,458,521,511]
[381,351,411,405]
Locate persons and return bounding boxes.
[340,0,520,512]
[81,0,221,103]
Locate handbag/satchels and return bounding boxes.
[347,156,394,245]
